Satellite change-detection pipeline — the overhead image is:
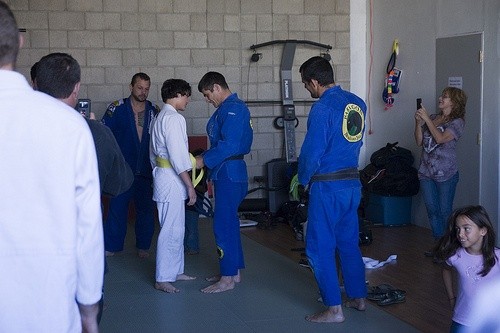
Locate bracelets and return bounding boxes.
[448,297,456,301]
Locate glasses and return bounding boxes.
[440,95,450,98]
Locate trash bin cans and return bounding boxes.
[363,193,411,226]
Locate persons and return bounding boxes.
[297,57,367,323]
[413,86,466,264]
[435,205,500,333]
[29,54,254,295]
[0,0,105,333]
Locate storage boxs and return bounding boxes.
[364,193,412,228]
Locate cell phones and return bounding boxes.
[416,98,422,110]
[78,99,91,119]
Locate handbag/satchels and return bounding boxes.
[369,142,414,172]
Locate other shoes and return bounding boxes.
[432,248,454,264]
[370,287,406,305]
[425,248,437,257]
